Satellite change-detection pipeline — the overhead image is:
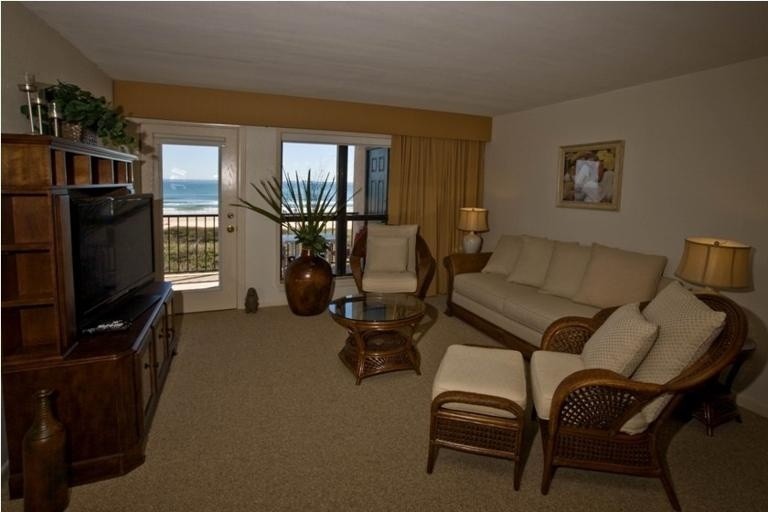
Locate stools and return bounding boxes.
[426,342,533,492]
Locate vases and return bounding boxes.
[17,386,72,511]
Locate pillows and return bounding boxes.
[581,297,660,381]
[366,235,410,272]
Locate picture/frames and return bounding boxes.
[555,140,624,210]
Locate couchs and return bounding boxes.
[443,233,677,360]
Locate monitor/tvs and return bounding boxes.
[70,193,164,334]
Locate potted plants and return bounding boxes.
[228,166,368,317]
[40,81,134,153]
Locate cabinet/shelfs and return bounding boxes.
[2,284,177,497]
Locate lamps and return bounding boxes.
[457,205,488,255]
[672,235,756,301]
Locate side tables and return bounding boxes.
[700,335,756,437]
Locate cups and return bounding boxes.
[47,101,63,138]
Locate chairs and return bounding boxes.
[349,218,437,309]
[527,277,748,512]
